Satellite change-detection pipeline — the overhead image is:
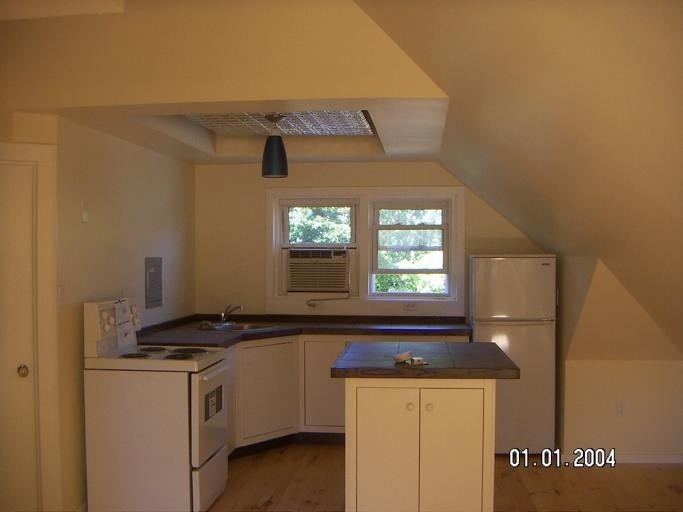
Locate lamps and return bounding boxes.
[261,114,288,179]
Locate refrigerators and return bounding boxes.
[467,252,560,459]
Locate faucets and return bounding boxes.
[221,305,244,322]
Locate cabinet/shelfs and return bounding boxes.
[226,335,470,460]
[343,379,495,511]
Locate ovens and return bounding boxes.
[81,358,232,512]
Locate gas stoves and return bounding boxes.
[83,343,229,375]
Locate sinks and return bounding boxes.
[214,323,280,332]
